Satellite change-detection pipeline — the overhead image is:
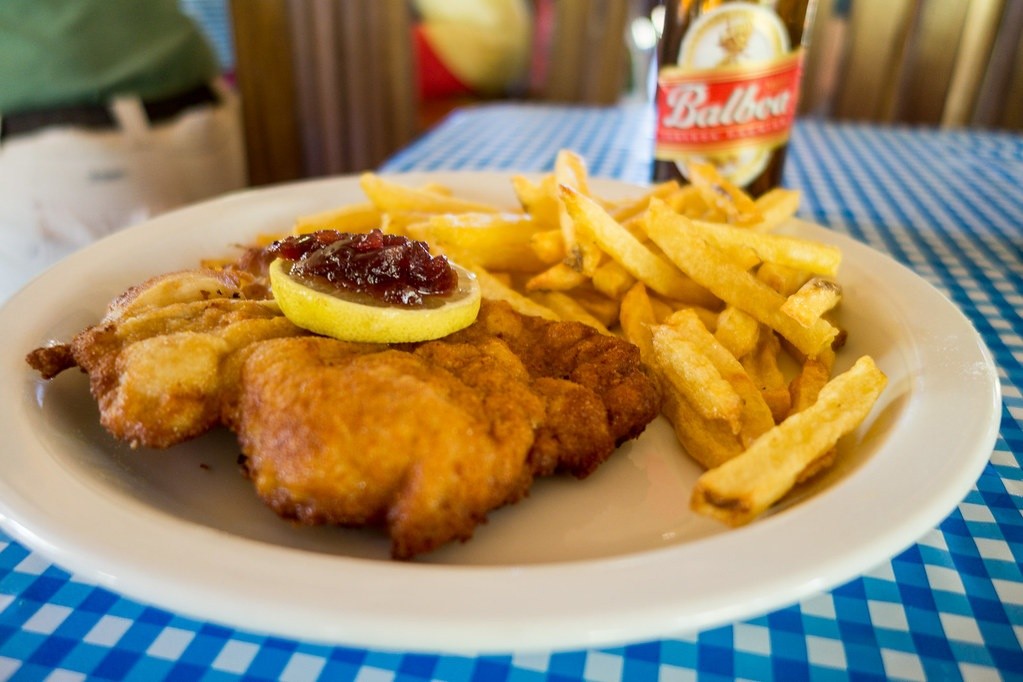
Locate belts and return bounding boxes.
[0,80,220,143]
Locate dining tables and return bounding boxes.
[0,104,1023,682]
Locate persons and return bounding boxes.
[0,0,249,315]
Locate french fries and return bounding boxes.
[284,146,887,520]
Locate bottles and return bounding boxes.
[648,1,806,197]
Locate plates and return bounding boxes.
[0,174,1004,657]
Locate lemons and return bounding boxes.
[269,251,482,342]
